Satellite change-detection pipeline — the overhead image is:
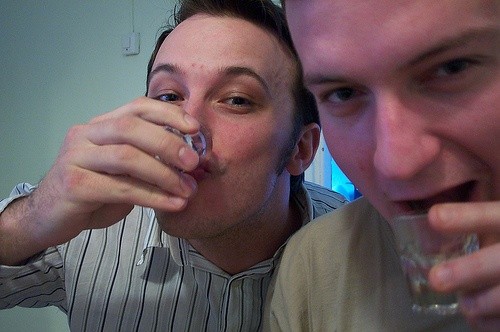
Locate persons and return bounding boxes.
[0,0,352,332]
[263,0,500,332]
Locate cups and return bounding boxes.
[155,119,208,172]
[391,210,479,315]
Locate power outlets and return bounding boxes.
[122,32,140,55]
